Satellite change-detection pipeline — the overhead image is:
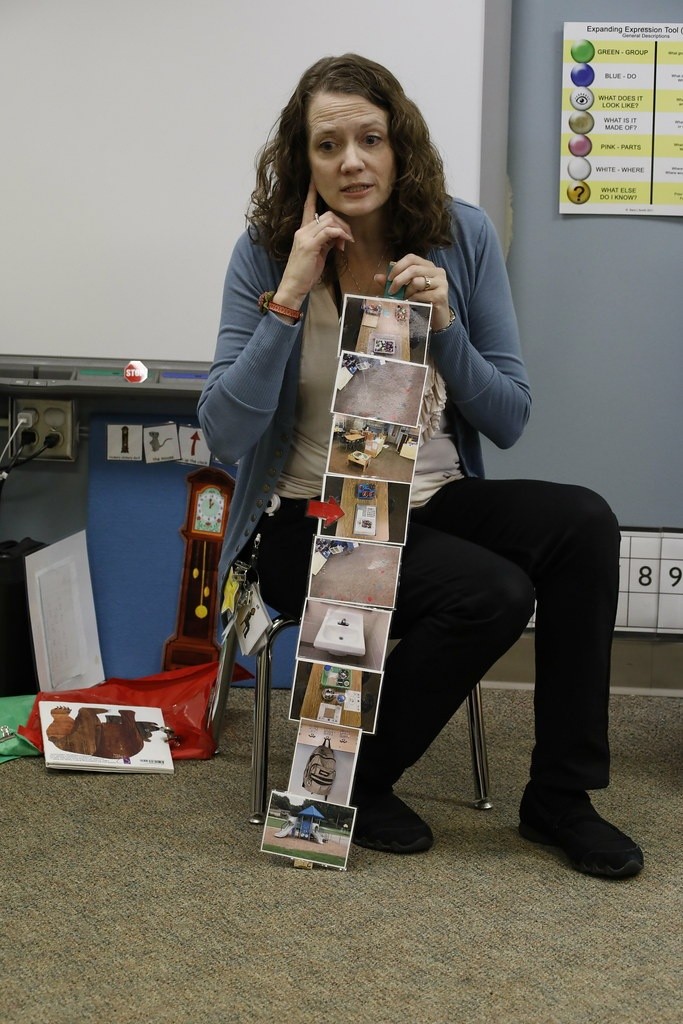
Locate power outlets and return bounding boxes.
[12,400,73,460]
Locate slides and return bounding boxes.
[312,832,324,844]
[274,826,294,838]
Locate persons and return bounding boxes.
[197,52,645,882]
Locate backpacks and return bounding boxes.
[302,738,337,802]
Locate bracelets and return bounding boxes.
[258,290,300,319]
[434,308,455,334]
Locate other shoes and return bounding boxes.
[518,780,644,879]
[349,790,434,855]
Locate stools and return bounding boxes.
[205,577,492,825]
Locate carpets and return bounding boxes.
[0,680,683,1023]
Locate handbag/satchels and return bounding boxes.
[16,659,219,762]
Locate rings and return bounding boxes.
[315,213,319,223]
[423,276,430,290]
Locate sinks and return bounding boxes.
[313,607,366,656]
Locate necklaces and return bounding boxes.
[340,248,383,296]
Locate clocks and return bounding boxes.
[161,464,236,673]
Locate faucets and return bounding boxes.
[341,618,347,625]
